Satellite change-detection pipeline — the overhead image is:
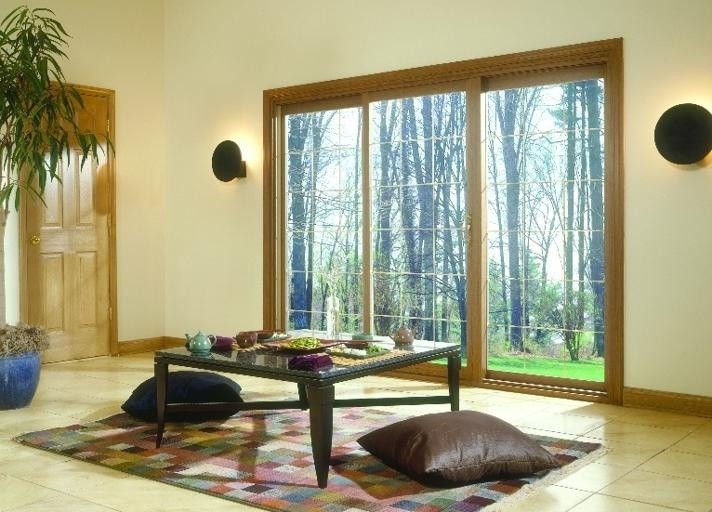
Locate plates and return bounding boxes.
[262,337,342,351]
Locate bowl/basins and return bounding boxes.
[344,342,368,349]
[235,333,257,348]
[258,333,274,339]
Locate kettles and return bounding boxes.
[185,331,218,353]
[389,326,418,346]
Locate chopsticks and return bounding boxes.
[251,329,285,332]
[337,339,384,342]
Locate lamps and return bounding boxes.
[211,141,246,182]
[654,103,712,164]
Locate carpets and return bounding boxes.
[14,393,603,512]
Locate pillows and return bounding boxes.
[122,371,246,420]
[356,410,563,485]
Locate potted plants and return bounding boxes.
[0,4,117,412]
